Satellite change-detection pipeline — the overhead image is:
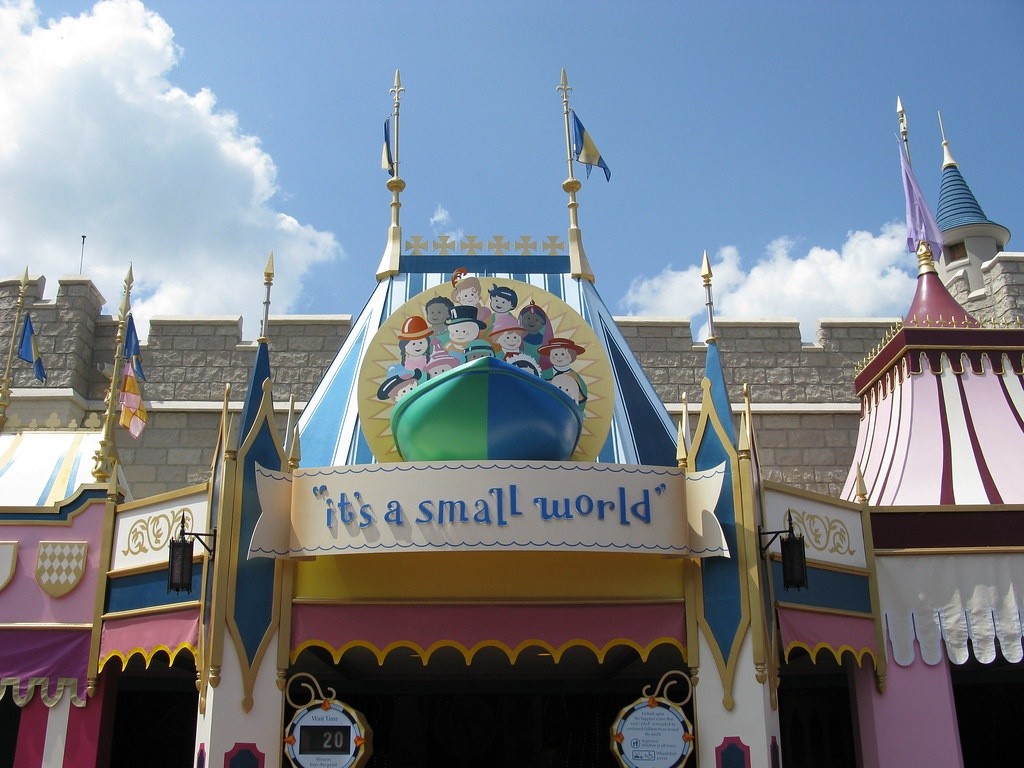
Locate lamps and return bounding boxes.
[165,511,218,597]
[757,506,810,592]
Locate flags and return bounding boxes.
[103,314,148,439]
[566,105,611,182]
[376,117,407,184]
[17,313,48,382]
[899,138,945,265]
